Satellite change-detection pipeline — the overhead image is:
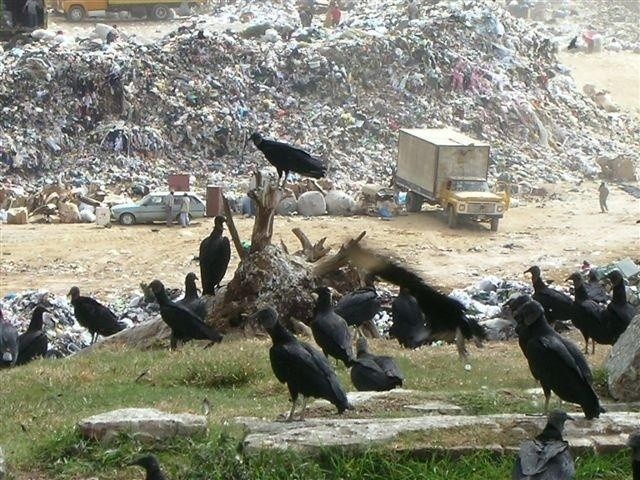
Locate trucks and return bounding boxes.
[396,128,506,232]
[56,0,207,22]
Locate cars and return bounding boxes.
[110,192,205,226]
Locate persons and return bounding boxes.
[166,191,175,228]
[599,182,610,212]
[298,3,342,28]
[568,32,622,53]
[26,23,121,48]
[180,193,191,228]
[165,25,206,40]
